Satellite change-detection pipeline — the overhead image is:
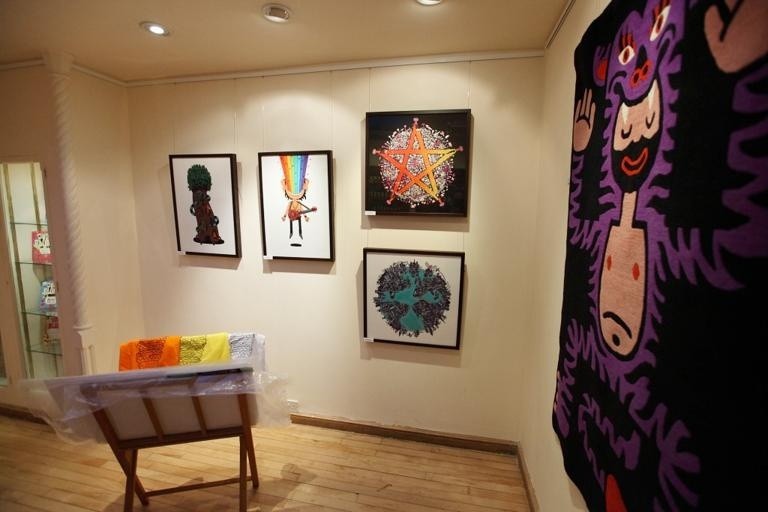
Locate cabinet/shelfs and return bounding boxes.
[10,219,62,356]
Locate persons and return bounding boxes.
[282,176,311,240]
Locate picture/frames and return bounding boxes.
[363,247,465,350]
[258,150,335,262]
[169,153,242,258]
[365,109,471,217]
